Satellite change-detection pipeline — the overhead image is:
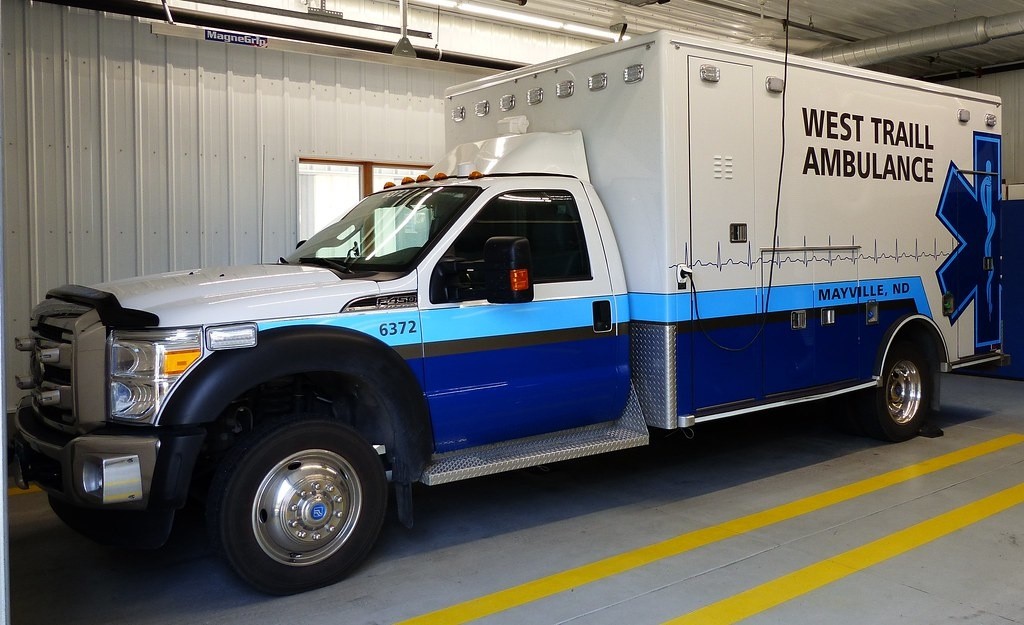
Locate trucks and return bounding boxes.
[12,29,1010,588]
